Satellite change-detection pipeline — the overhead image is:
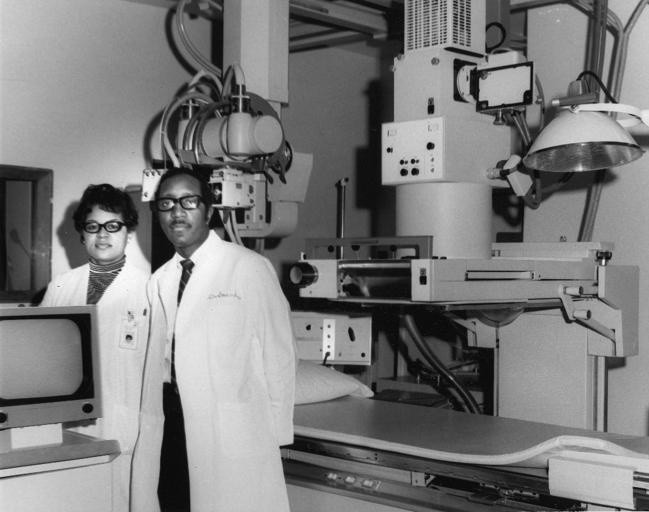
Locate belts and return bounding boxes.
[165,383,179,395]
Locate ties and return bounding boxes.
[171,259,194,386]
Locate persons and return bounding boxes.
[31,181,151,512]
[128,166,298,512]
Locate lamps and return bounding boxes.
[523,80,649,172]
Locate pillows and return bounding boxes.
[295,361,373,404]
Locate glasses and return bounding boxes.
[83,221,127,233]
[154,195,207,212]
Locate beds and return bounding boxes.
[285,400,649,512]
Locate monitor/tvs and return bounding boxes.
[0,303,122,432]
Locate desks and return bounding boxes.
[0,428,121,512]
